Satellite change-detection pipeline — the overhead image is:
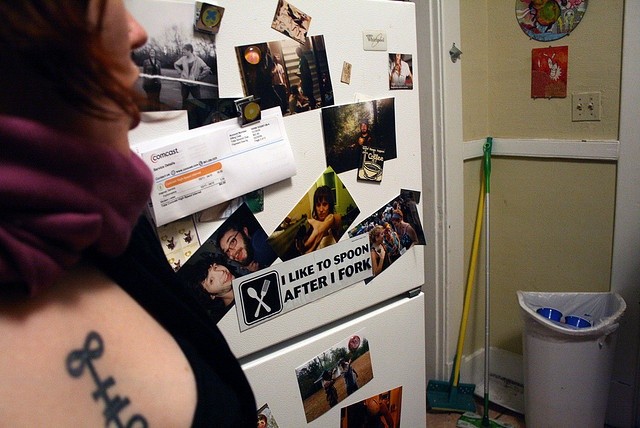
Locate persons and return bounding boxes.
[294,47,317,110]
[279,185,343,254]
[364,212,392,231]
[367,397,394,428]
[174,44,212,109]
[393,201,400,210]
[354,118,375,167]
[382,222,402,264]
[321,367,338,408]
[392,209,419,250]
[188,259,237,311]
[142,48,161,111]
[370,225,392,277]
[403,194,425,244]
[390,54,413,88]
[265,53,290,116]
[1,0,258,428]
[383,207,394,222]
[338,357,358,396]
[216,224,277,274]
[258,415,267,428]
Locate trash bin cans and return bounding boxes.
[516,289,627,428]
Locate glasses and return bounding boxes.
[225,230,240,255]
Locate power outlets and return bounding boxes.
[572,91,602,122]
